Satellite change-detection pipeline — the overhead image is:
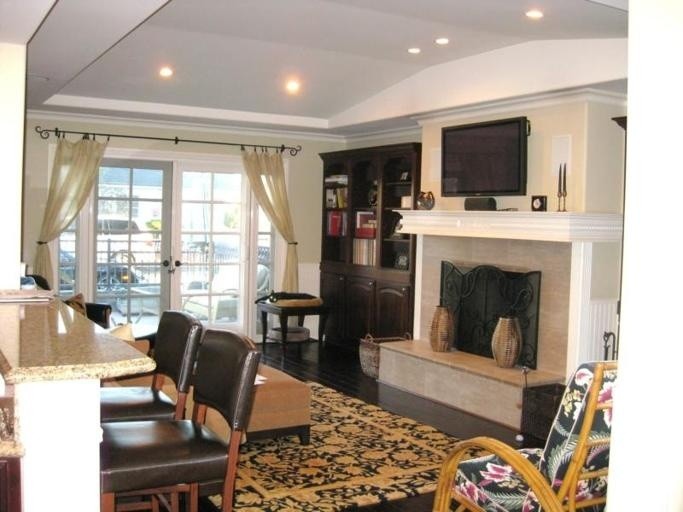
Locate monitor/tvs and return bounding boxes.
[440,115,527,197]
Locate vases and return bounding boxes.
[430,305,455,353]
[491,315,522,368]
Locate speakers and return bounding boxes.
[531,195,546,211]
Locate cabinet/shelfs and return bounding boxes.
[319,141,420,354]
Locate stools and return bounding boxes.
[245,362,312,446]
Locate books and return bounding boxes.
[325,175,348,236]
[0,288,57,302]
[401,196,412,208]
[352,211,376,266]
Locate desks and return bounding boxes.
[258,301,330,361]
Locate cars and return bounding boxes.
[59,215,156,290]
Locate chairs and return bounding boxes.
[98,311,261,511]
[432,359,618,511]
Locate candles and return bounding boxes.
[558,163,566,193]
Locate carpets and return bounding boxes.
[207,381,467,511]
